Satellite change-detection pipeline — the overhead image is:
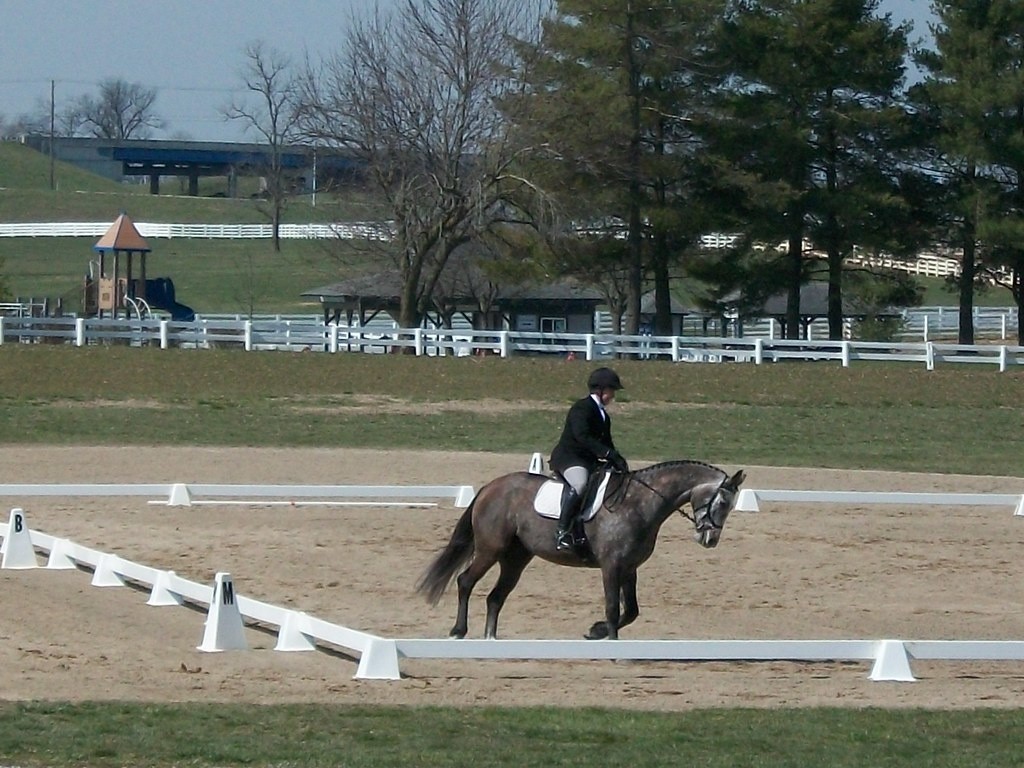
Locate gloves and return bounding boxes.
[603,450,629,471]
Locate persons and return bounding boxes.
[549,368,628,552]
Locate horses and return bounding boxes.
[419,460,747,642]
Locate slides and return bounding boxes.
[148,278,195,345]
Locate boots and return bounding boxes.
[555,487,585,547]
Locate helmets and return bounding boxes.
[588,367,625,389]
